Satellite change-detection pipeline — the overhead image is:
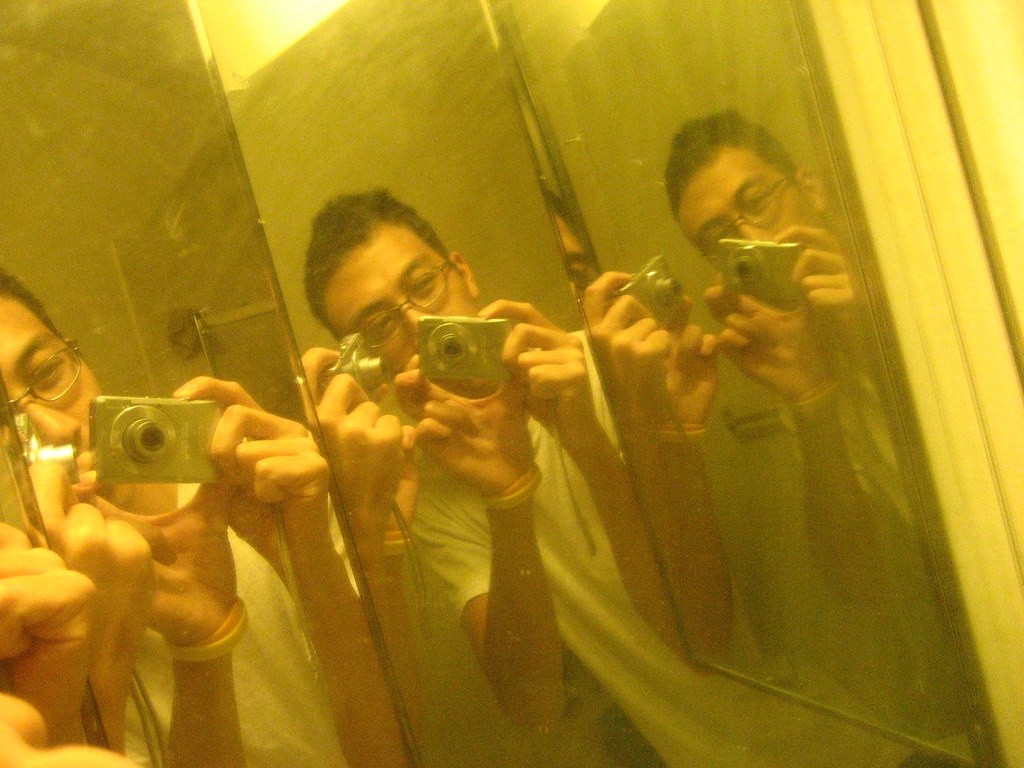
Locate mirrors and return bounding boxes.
[0,1,1024,768]
[481,0,997,767]
[184,0,1024,768]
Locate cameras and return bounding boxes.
[13,411,79,476]
[718,238,804,304]
[88,394,225,485]
[316,332,392,398]
[613,253,686,332]
[416,315,515,383]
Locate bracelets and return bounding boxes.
[383,531,411,557]
[797,379,839,409]
[652,424,707,445]
[167,596,249,663]
[480,462,543,508]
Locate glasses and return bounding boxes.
[701,175,796,260]
[0,337,82,429]
[364,258,450,348]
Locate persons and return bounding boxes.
[1,269,410,768]
[297,185,913,767]
[1,522,140,768]
[28,463,153,754]
[296,346,417,571]
[581,270,721,662]
[666,111,966,742]
